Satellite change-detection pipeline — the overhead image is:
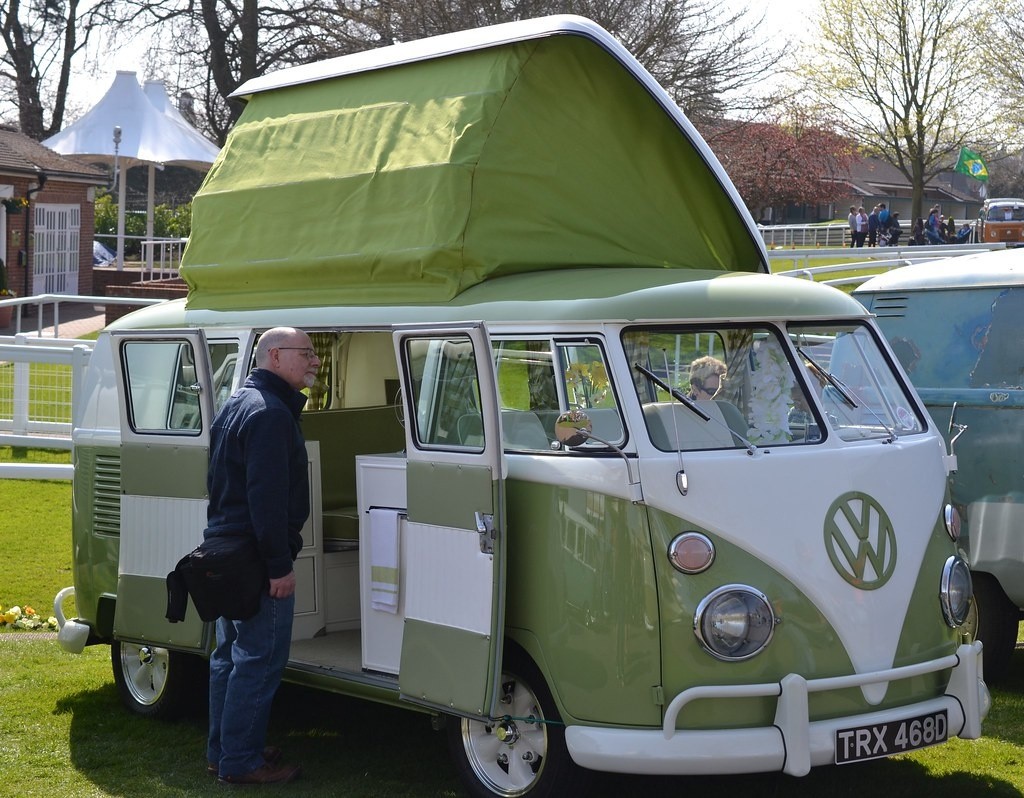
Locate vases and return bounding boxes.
[5,206,24,214]
[0,296,13,329]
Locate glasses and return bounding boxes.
[268,348,318,360]
[697,384,718,395]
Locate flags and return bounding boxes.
[952,145,990,182]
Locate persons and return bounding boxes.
[868,206,879,247]
[674,356,727,402]
[877,203,889,247]
[925,208,939,245]
[939,204,971,244]
[934,203,940,210]
[856,207,868,247]
[913,217,923,245]
[848,205,858,248]
[889,212,903,246]
[201,326,323,788]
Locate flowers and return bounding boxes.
[0,605,60,633]
[2,196,32,210]
[0,289,18,298]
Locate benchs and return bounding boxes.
[300,404,405,542]
[642,401,749,452]
[457,407,622,449]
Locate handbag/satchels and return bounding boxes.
[164,534,271,624]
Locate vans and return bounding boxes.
[973,196,1024,246]
[820,245,1024,695]
[50,13,992,796]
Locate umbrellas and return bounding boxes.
[38,70,221,270]
